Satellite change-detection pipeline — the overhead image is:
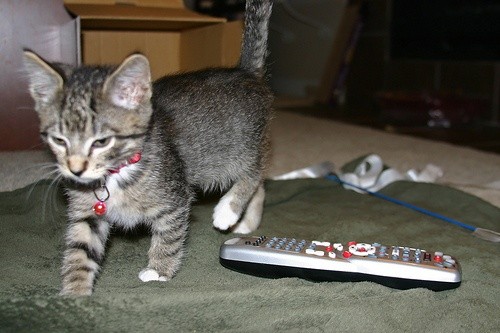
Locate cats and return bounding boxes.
[20,0,276,297]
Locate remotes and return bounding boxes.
[219,234,462,292]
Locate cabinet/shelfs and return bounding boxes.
[60,0,243,82]
